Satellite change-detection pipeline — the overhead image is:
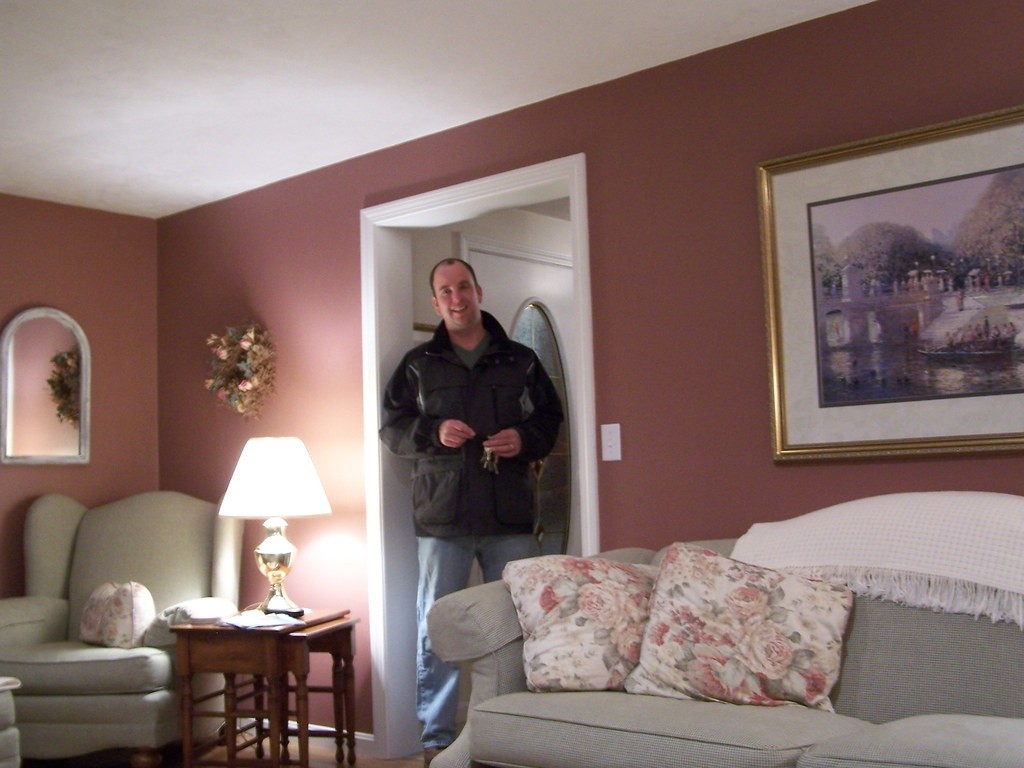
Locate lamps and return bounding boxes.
[219,436,332,616]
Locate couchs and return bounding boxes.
[427,490,1024,768]
[0,489,246,768]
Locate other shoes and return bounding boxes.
[424,747,447,768]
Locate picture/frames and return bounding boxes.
[754,105,1024,464]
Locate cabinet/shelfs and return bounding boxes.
[168,609,362,768]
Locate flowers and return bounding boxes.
[205,322,276,415]
[46,346,82,427]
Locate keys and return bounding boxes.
[480,446,498,475]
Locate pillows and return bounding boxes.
[78,580,155,649]
[624,541,854,715]
[501,554,655,693]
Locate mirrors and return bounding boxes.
[0,307,92,466]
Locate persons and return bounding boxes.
[379,257,566,768]
